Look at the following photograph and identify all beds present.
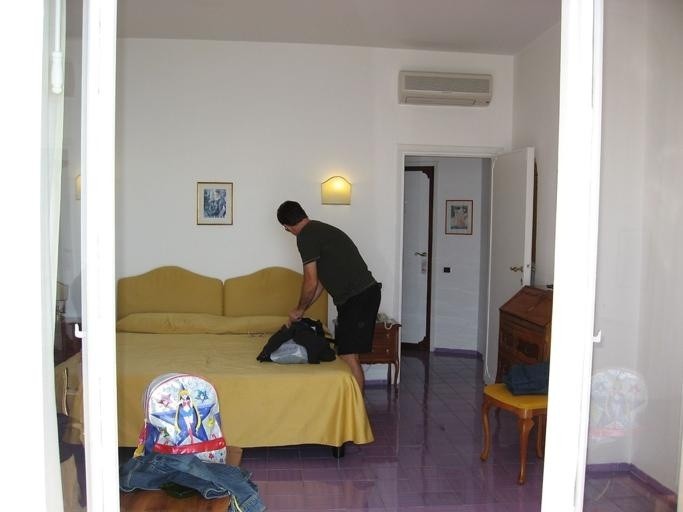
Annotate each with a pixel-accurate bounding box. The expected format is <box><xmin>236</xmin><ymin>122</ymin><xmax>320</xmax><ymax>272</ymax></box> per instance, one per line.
<box><xmin>55</xmin><ymin>266</ymin><xmax>375</xmax><ymax>457</ymax></box>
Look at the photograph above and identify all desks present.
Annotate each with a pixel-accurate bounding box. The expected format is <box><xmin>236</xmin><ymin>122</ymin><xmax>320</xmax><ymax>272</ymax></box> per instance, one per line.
<box><xmin>119</xmin><ymin>445</ymin><xmax>242</xmax><ymax>512</ymax></box>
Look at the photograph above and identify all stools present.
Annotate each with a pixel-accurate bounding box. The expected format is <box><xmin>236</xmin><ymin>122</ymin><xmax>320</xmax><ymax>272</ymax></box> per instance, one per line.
<box><xmin>481</xmin><ymin>384</ymin><xmax>548</xmax><ymax>485</ymax></box>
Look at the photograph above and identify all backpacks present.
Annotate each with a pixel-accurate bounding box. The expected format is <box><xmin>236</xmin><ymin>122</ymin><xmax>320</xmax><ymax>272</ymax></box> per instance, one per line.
<box><xmin>134</xmin><ymin>373</ymin><xmax>227</xmax><ymax>471</ymax></box>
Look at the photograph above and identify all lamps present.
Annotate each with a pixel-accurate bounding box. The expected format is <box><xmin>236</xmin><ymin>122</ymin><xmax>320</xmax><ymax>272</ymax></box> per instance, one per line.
<box><xmin>321</xmin><ymin>176</ymin><xmax>351</xmax><ymax>206</ymax></box>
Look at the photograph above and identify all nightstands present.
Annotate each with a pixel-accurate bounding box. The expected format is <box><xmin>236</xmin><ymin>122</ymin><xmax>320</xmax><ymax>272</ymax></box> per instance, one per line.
<box><xmin>333</xmin><ymin>317</ymin><xmax>401</xmax><ymax>398</ymax></box>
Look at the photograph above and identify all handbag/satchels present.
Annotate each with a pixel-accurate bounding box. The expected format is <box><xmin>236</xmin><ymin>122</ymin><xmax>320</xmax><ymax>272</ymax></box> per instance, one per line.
<box><xmin>501</xmin><ymin>364</ymin><xmax>548</xmax><ymax>395</ymax></box>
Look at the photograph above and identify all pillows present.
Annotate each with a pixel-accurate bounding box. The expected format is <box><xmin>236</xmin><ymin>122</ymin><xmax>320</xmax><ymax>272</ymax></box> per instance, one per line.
<box><xmin>116</xmin><ymin>312</ymin><xmax>333</xmax><ymax>341</ymax></box>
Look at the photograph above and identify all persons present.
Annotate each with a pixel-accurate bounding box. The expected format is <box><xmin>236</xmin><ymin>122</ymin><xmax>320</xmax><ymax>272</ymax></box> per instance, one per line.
<box><xmin>275</xmin><ymin>199</ymin><xmax>383</xmax><ymax>399</ymax></box>
<box><xmin>208</xmin><ymin>191</ymin><xmax>226</xmax><ymax>218</ymax></box>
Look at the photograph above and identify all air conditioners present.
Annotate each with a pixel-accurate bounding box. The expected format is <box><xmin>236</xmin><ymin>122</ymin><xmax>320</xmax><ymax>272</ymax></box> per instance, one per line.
<box><xmin>397</xmin><ymin>72</ymin><xmax>496</xmax><ymax>106</ymax></box>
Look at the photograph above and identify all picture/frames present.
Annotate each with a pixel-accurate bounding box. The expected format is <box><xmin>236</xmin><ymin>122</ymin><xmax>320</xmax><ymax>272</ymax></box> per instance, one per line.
<box><xmin>445</xmin><ymin>199</ymin><xmax>473</xmax><ymax>236</ymax></box>
<box><xmin>196</xmin><ymin>181</ymin><xmax>234</xmax><ymax>225</ymax></box>
